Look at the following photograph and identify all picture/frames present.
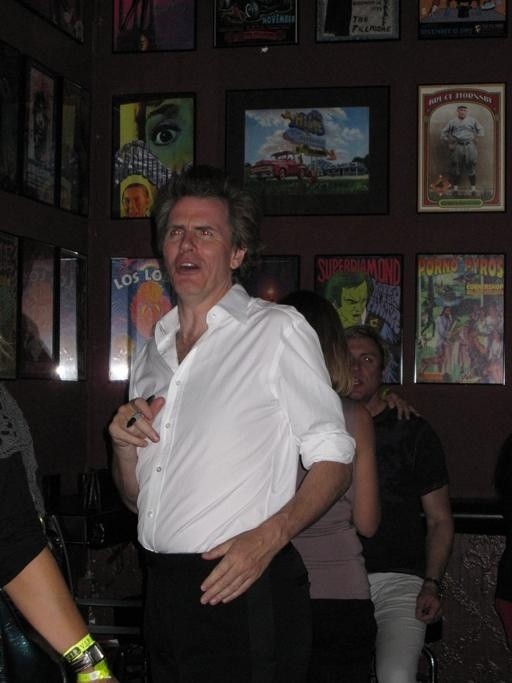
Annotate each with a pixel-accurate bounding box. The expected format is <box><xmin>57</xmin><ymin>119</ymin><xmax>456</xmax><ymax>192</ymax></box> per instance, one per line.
<box><xmin>21</xmin><ymin>54</ymin><xmax>57</xmax><ymax>210</ymax></box>
<box><xmin>222</xmin><ymin>83</ymin><xmax>394</xmax><ymax>219</ymax></box>
<box><xmin>411</xmin><ymin>250</ymin><xmax>507</xmax><ymax>386</ymax></box>
<box><xmin>414</xmin><ymin>81</ymin><xmax>507</xmax><ymax>217</ymax></box>
<box><xmin>58</xmin><ymin>245</ymin><xmax>90</xmax><ymax>382</ymax></box>
<box><xmin>228</xmin><ymin>253</ymin><xmax>303</xmax><ymax>297</ymax></box>
<box><xmin>57</xmin><ymin>72</ymin><xmax>94</xmax><ymax>220</ymax></box>
<box><xmin>212</xmin><ymin>2</ymin><xmax>300</xmax><ymax>50</ymax></box>
<box><xmin>19</xmin><ymin>234</ymin><xmax>57</xmax><ymax>382</ymax></box>
<box><xmin>107</xmin><ymin>89</ymin><xmax>199</xmax><ymax>222</ymax></box>
<box><xmin>416</xmin><ymin>2</ymin><xmax>510</xmax><ymax>42</ymax></box>
<box><xmin>312</xmin><ymin>252</ymin><xmax>407</xmax><ymax>386</ymax></box>
<box><xmin>106</xmin><ymin>255</ymin><xmax>179</xmax><ymax>385</ymax></box>
<box><xmin>314</xmin><ymin>2</ymin><xmax>403</xmax><ymax>44</ymax></box>
<box><xmin>110</xmin><ymin>2</ymin><xmax>198</xmax><ymax>55</ymax></box>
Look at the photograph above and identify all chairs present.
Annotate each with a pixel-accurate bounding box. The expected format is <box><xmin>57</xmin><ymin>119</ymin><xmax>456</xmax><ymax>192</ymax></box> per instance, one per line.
<box><xmin>41</xmin><ymin>469</ymin><xmax>442</xmax><ymax>683</ymax></box>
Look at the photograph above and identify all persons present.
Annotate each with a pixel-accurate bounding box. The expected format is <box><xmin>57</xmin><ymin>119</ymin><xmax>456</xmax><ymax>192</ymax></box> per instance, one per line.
<box><xmin>0</xmin><ymin>384</ymin><xmax>120</xmax><ymax>682</ymax></box>
<box><xmin>327</xmin><ymin>270</ymin><xmax>396</xmax><ymax>381</ymax></box>
<box><xmin>434</xmin><ymin>286</ymin><xmax>505</xmax><ymax>384</ymax></box>
<box><xmin>107</xmin><ymin>176</ymin><xmax>358</xmax><ymax>683</ymax></box>
<box><xmin>490</xmin><ymin>429</ymin><xmax>511</xmax><ymax>651</ymax></box>
<box><xmin>341</xmin><ymin>324</ymin><xmax>455</xmax><ymax>682</ymax></box>
<box><xmin>441</xmin><ymin>105</ymin><xmax>486</xmax><ymax>198</ymax></box>
<box><xmin>117</xmin><ymin>99</ymin><xmax>194</xmax><ymax>216</ymax></box>
<box><xmin>278</xmin><ymin>291</ymin><xmax>421</xmax><ymax>683</ymax></box>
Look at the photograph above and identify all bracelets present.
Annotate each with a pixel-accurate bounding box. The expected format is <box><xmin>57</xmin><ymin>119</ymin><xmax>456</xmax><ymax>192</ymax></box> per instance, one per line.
<box><xmin>71</xmin><ymin>641</ymin><xmax>105</xmax><ymax>671</ymax></box>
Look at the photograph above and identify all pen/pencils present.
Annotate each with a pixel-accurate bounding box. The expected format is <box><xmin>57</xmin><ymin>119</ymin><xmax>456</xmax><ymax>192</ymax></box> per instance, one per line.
<box><xmin>126</xmin><ymin>395</ymin><xmax>155</xmax><ymax>428</ymax></box>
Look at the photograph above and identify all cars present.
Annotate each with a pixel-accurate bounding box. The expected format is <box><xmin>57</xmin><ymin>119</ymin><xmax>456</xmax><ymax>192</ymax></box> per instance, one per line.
<box><xmin>253</xmin><ymin>150</ymin><xmax>308</xmax><ymax>181</ymax></box>
